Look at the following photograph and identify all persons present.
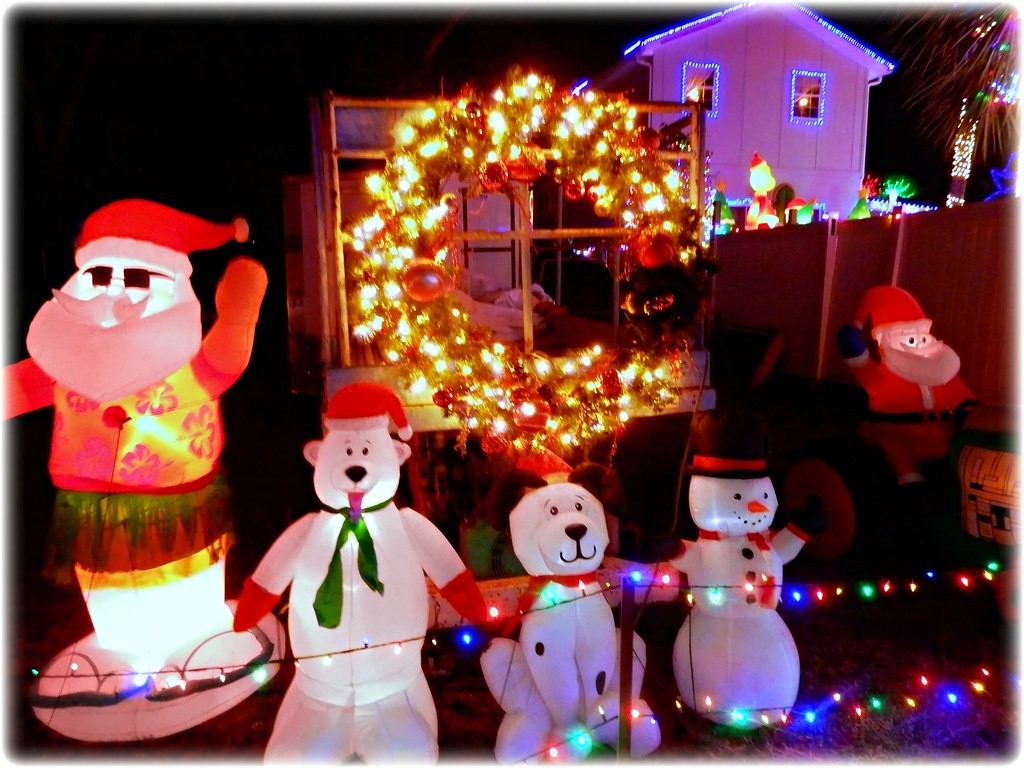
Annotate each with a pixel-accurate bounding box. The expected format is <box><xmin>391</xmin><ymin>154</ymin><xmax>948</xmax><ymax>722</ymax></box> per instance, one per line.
<box><xmin>6</xmin><ymin>201</ymin><xmax>267</xmax><ymax>689</ymax></box>
<box><xmin>837</xmin><ymin>285</ymin><xmax>975</xmax><ymax>504</ymax></box>
